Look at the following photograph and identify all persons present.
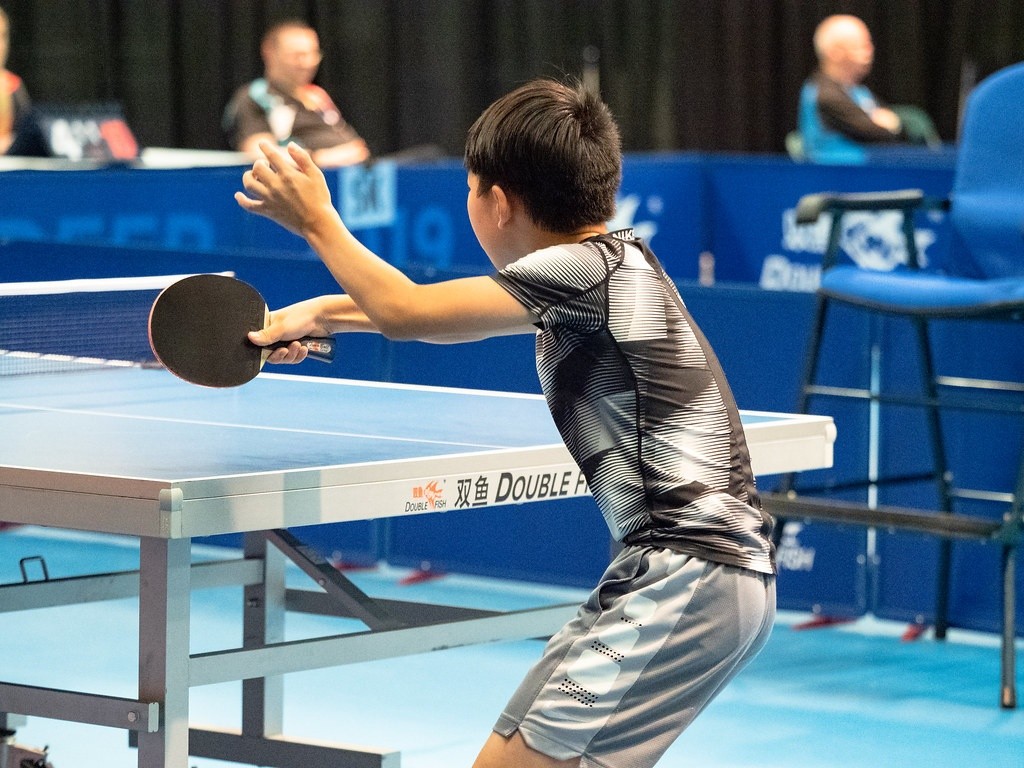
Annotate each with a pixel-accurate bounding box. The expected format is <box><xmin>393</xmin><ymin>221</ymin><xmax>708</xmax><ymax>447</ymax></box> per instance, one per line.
<box><xmin>799</xmin><ymin>14</ymin><xmax>910</xmax><ymax>162</ymax></box>
<box><xmin>232</xmin><ymin>80</ymin><xmax>776</xmax><ymax>768</ymax></box>
<box><xmin>0</xmin><ymin>8</ymin><xmax>32</xmax><ymax>155</ymax></box>
<box><xmin>224</xmin><ymin>18</ymin><xmax>370</xmax><ymax>169</ymax></box>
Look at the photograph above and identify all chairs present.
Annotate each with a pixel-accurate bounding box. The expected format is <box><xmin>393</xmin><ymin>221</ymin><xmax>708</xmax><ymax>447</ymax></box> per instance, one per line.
<box><xmin>760</xmin><ymin>61</ymin><xmax>1024</xmax><ymax>711</ymax></box>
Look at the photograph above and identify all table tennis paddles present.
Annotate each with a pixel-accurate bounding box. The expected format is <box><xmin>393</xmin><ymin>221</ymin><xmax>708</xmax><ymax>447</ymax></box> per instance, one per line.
<box><xmin>146</xmin><ymin>270</ymin><xmax>337</xmax><ymax>389</ymax></box>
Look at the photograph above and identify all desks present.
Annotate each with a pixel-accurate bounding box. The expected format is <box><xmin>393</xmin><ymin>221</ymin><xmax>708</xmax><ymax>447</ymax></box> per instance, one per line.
<box><xmin>0</xmin><ymin>271</ymin><xmax>840</xmax><ymax>768</ymax></box>
